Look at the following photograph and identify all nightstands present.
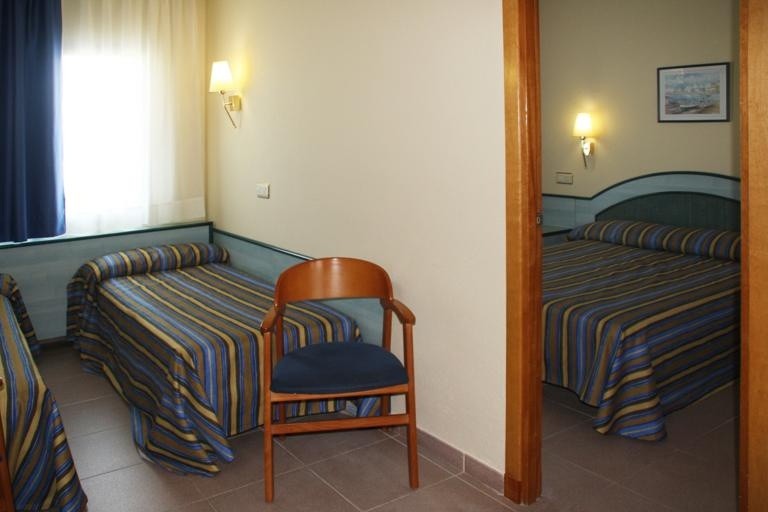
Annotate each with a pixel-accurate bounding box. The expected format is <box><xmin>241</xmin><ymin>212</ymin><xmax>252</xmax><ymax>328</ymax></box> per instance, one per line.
<box><xmin>542</xmin><ymin>225</ymin><xmax>572</xmax><ymax>245</ymax></box>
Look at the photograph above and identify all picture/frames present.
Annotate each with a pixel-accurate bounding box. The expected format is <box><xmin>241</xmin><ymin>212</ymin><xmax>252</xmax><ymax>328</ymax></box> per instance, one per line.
<box><xmin>657</xmin><ymin>61</ymin><xmax>730</xmax><ymax>123</ymax></box>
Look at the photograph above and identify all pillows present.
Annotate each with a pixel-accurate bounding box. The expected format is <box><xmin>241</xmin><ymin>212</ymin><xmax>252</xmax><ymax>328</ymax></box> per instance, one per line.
<box><xmin>567</xmin><ymin>219</ymin><xmax>741</xmax><ymax>261</ymax></box>
<box><xmin>79</xmin><ymin>241</ymin><xmax>230</xmax><ymax>282</ymax></box>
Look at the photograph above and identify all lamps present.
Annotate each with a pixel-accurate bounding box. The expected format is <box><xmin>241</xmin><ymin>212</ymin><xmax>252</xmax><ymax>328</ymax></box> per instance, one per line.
<box><xmin>209</xmin><ymin>60</ymin><xmax>240</xmax><ymax>129</ymax></box>
<box><xmin>572</xmin><ymin>111</ymin><xmax>593</xmax><ymax>168</ymax></box>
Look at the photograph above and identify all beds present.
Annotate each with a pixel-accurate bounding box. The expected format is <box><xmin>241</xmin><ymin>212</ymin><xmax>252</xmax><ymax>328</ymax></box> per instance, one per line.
<box><xmin>65</xmin><ymin>242</ymin><xmax>390</xmax><ymax>479</ymax></box>
<box><xmin>541</xmin><ymin>190</ymin><xmax>742</xmax><ymax>442</ymax></box>
<box><xmin>0</xmin><ymin>273</ymin><xmax>87</xmax><ymax>511</ymax></box>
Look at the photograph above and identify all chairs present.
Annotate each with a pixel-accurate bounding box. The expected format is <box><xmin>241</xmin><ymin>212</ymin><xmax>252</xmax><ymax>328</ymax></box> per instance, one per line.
<box><xmin>259</xmin><ymin>255</ymin><xmax>419</xmax><ymax>502</ymax></box>
